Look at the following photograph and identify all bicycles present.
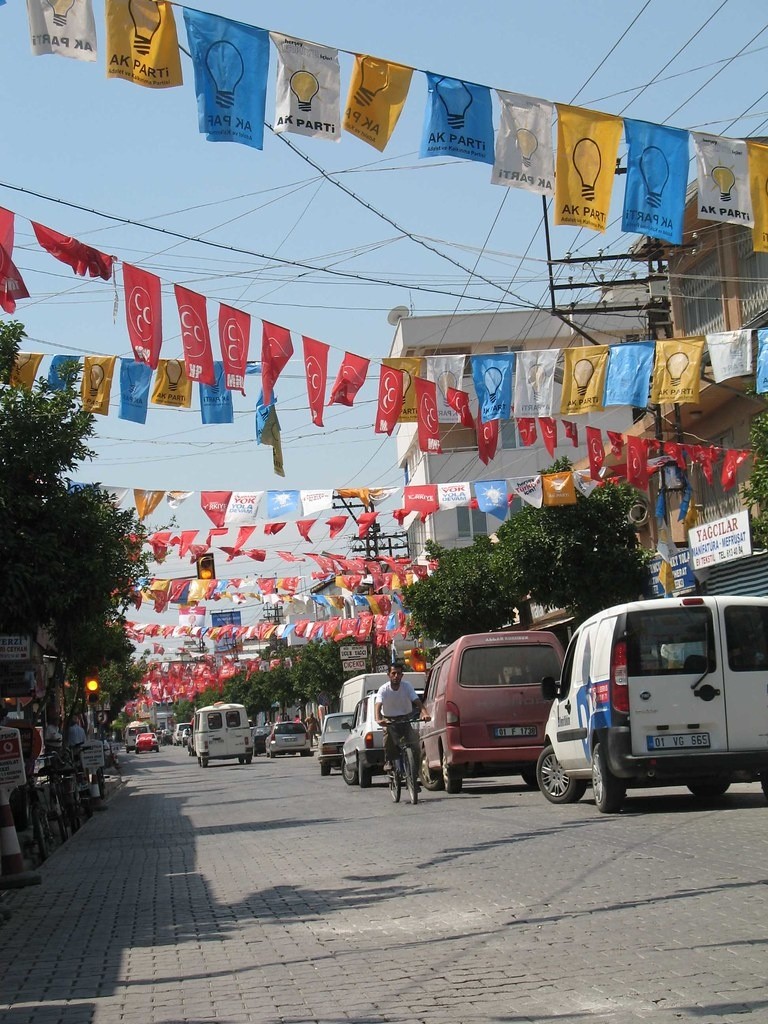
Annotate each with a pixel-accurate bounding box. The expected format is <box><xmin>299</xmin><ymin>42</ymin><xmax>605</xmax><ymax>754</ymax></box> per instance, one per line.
<box><xmin>21</xmin><ymin>738</ymin><xmax>110</xmax><ymax>865</ymax></box>
<box><xmin>379</xmin><ymin>718</ymin><xmax>429</xmax><ymax>806</ymax></box>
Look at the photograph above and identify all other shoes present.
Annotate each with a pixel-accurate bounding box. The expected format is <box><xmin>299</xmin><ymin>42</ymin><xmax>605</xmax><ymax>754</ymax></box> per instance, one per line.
<box><xmin>383</xmin><ymin>761</ymin><xmax>393</xmax><ymax>771</ymax></box>
<box><xmin>417</xmin><ymin>784</ymin><xmax>422</xmax><ymax>793</ymax></box>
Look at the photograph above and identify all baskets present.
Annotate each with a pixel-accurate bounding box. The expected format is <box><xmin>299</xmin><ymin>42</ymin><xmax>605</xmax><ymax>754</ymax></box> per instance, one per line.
<box><xmin>388</xmin><ymin>721</ymin><xmax>419</xmax><ymax>745</ymax></box>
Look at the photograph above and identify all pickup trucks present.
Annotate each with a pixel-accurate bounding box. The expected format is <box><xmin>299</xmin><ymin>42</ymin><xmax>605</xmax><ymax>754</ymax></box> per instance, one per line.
<box><xmin>133</xmin><ymin>733</ymin><xmax>160</xmax><ymax>753</ymax></box>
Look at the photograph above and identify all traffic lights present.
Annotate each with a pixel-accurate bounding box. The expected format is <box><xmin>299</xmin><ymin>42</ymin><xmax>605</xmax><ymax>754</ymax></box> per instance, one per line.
<box><xmin>86</xmin><ymin>675</ymin><xmax>100</xmax><ymax>707</ymax></box>
<box><xmin>197</xmin><ymin>553</ymin><xmax>216</xmax><ymax>579</ymax></box>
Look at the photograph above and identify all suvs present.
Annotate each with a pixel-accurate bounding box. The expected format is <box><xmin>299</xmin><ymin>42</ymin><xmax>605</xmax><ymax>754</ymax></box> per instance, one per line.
<box><xmin>340</xmin><ymin>691</ymin><xmax>425</xmax><ymax>788</ymax></box>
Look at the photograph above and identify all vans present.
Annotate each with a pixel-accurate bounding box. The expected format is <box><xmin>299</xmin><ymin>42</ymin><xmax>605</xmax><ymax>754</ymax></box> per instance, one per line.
<box><xmin>339</xmin><ymin>672</ymin><xmax>427</xmax><ymax>715</ymax></box>
<box><xmin>536</xmin><ymin>595</ymin><xmax>768</xmax><ymax>813</ymax></box>
<box><xmin>418</xmin><ymin>630</ymin><xmax>569</xmax><ymax>794</ymax></box>
<box><xmin>189</xmin><ymin>704</ymin><xmax>254</xmax><ymax>768</ymax></box>
<box><xmin>124</xmin><ymin>724</ymin><xmax>152</xmax><ymax>754</ymax></box>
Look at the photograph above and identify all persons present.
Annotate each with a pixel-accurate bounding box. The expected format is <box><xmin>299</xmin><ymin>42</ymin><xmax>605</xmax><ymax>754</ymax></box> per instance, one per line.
<box><xmin>45</xmin><ymin>714</ymin><xmax>86</xmax><ymax>767</ymax></box>
<box><xmin>375</xmin><ymin>663</ymin><xmax>431</xmax><ymax>793</ymax></box>
<box><xmin>293</xmin><ymin>712</ymin><xmax>322</xmax><ymax>745</ymax></box>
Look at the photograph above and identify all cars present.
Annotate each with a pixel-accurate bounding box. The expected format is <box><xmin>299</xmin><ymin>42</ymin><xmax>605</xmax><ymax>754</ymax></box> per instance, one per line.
<box><xmin>264</xmin><ymin>721</ymin><xmax>312</xmax><ymax>758</ymax></box>
<box><xmin>318</xmin><ymin>712</ymin><xmax>356</xmax><ymax>776</ymax></box>
<box><xmin>163</xmin><ymin>722</ymin><xmax>196</xmax><ymax>757</ymax></box>
<box><xmin>252</xmin><ymin>726</ymin><xmax>273</xmax><ymax>758</ymax></box>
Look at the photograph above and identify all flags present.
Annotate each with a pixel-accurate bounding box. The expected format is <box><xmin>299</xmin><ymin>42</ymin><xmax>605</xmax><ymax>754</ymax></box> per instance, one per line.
<box><xmin>692</xmin><ymin>131</ymin><xmax>768</xmax><ymax>252</ymax></box>
<box><xmin>268</xmin><ymin>32</ymin><xmax>341</xmax><ymax>142</ymax></box>
<box><xmin>26</xmin><ymin>0</ymin><xmax>97</xmax><ymax>61</ymax></box>
<box><xmin>418</xmin><ymin>74</ymin><xmax>495</xmax><ymax>165</ymax></box>
<box><xmin>105</xmin><ymin>0</ymin><xmax>183</xmax><ymax>88</ymax></box>
<box><xmin>554</xmin><ymin>103</ymin><xmax>623</xmax><ymax>234</ymax></box>
<box><xmin>621</xmin><ymin>118</ymin><xmax>690</xmax><ymax>245</ymax></box>
<box><xmin>0</xmin><ymin>208</ymin><xmax>768</xmax><ymax>716</ymax></box>
<box><xmin>490</xmin><ymin>91</ymin><xmax>554</xmax><ymax>197</ymax></box>
<box><xmin>182</xmin><ymin>6</ymin><xmax>270</xmax><ymax>150</ymax></box>
<box><xmin>340</xmin><ymin>53</ymin><xmax>413</xmax><ymax>152</ymax></box>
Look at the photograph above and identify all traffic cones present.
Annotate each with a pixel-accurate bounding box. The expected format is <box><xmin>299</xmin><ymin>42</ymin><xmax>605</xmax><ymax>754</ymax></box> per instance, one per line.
<box><xmin>114</xmin><ymin>755</ymin><xmax>122</xmax><ymax>767</ymax></box>
